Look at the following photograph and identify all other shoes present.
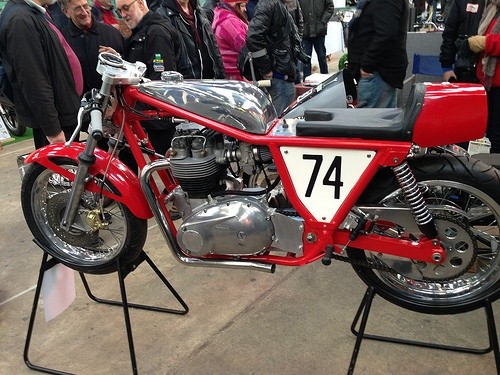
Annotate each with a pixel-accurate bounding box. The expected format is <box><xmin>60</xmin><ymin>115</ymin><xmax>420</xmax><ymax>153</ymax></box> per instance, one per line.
<box><xmin>100</xmin><ymin>211</ymin><xmax>112</xmax><ymax>224</ymax></box>
<box><xmin>91</xmin><ymin>236</ymin><xmax>104</xmax><ymax>247</ymax></box>
<box><xmin>96</xmin><ymin>194</ymin><xmax>114</xmax><ymax>208</ymax></box>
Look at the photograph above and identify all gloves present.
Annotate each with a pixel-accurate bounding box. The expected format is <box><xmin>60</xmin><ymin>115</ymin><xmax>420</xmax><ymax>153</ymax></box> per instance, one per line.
<box><xmin>468</xmin><ymin>35</ymin><xmax>486</xmax><ymax>53</ymax></box>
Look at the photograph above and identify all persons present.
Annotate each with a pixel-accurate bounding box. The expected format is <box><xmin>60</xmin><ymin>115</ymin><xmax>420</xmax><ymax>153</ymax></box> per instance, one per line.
<box><xmin>298</xmin><ymin>0</ymin><xmax>335</xmax><ymax>83</ymax></box>
<box><xmin>0</xmin><ymin>0</ymin><xmax>84</xmax><ymax>150</ymax></box>
<box><xmin>90</xmin><ymin>0</ymin><xmax>313</xmax><ymax>116</ymax></box>
<box><xmin>467</xmin><ymin>0</ymin><xmax>500</xmax><ymax>153</ymax></box>
<box><xmin>347</xmin><ymin>0</ymin><xmax>410</xmax><ymax>108</ymax></box>
<box><xmin>57</xmin><ymin>0</ymin><xmax>126</xmax><ymax>133</ymax></box>
<box><xmin>438</xmin><ymin>0</ymin><xmax>486</xmax><ymax>82</ymax></box>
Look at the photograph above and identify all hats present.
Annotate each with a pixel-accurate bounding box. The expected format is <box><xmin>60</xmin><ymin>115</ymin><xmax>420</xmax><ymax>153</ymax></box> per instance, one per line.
<box><xmin>221</xmin><ymin>0</ymin><xmax>248</xmax><ymax>7</ymax></box>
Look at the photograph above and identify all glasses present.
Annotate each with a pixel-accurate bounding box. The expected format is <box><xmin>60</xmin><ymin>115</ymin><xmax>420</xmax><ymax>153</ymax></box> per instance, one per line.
<box><xmin>115</xmin><ymin>0</ymin><xmax>137</xmax><ymax>15</ymax></box>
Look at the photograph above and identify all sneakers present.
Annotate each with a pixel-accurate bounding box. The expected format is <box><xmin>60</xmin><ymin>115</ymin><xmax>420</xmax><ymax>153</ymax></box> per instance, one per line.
<box><xmin>168</xmin><ymin>203</ymin><xmax>182</xmax><ymax>220</ymax></box>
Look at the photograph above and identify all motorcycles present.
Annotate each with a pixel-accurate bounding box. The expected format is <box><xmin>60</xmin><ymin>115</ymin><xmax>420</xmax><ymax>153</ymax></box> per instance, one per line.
<box><xmin>18</xmin><ymin>46</ymin><xmax>500</xmax><ymax>316</ymax></box>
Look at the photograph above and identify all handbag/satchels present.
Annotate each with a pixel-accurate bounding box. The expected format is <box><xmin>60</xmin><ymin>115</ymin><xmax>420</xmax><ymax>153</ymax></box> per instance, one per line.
<box><xmin>454</xmin><ymin>35</ymin><xmax>478</xmax><ymax>83</ymax></box>
<box><xmin>236</xmin><ymin>46</ymin><xmax>261</xmax><ymax>81</ymax></box>
